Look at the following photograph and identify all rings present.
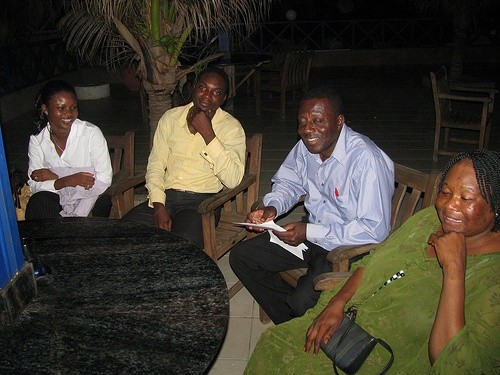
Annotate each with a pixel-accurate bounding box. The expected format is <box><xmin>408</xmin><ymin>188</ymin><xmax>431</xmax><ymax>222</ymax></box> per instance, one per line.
<box><xmin>35</xmin><ymin>177</ymin><xmax>37</xmax><ymax>179</ymax></box>
<box><xmin>87</xmin><ymin>185</ymin><xmax>89</xmax><ymax>188</ymax></box>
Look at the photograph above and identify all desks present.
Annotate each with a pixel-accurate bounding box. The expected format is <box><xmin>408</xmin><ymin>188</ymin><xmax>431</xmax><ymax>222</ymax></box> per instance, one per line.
<box><xmin>211</xmin><ymin>53</ymin><xmax>273</xmax><ymax>97</ymax></box>
<box><xmin>0</xmin><ymin>220</ymin><xmax>231</xmax><ymax>375</ymax></box>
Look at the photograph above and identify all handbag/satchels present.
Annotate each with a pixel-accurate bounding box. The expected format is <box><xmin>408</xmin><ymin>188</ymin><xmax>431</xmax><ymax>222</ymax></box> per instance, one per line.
<box><xmin>318</xmin><ymin>306</ymin><xmax>395</xmax><ymax>375</ymax></box>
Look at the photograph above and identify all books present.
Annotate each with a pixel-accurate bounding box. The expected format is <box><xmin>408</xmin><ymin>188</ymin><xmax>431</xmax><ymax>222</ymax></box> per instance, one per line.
<box><xmin>232</xmin><ymin>221</ymin><xmax>309</xmax><ymax>260</ymax></box>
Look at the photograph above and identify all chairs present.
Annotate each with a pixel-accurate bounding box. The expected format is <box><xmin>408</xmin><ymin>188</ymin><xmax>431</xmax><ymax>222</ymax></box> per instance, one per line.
<box><xmin>251</xmin><ymin>162</ymin><xmax>443</xmax><ymax>324</ymax></box>
<box><xmin>104</xmin><ymin>132</ymin><xmax>262</xmax><ymax>299</ymax></box>
<box><xmin>177</xmin><ymin>43</ymin><xmax>314</xmax><ymax>121</ymax></box>
<box><xmin>87</xmin><ymin>131</ymin><xmax>136</xmax><ymax>218</ymax></box>
<box><xmin>429</xmin><ymin>64</ymin><xmax>498</xmax><ymax>162</ymax></box>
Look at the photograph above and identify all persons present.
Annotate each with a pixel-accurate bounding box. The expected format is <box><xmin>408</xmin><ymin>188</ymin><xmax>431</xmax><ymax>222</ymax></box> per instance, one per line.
<box><xmin>25</xmin><ymin>84</ymin><xmax>114</xmax><ymax>258</ymax></box>
<box><xmin>122</xmin><ymin>67</ymin><xmax>247</xmax><ymax>248</ymax></box>
<box><xmin>242</xmin><ymin>152</ymin><xmax>500</xmax><ymax>375</ymax></box>
<box><xmin>230</xmin><ymin>88</ymin><xmax>395</xmax><ymax>326</ymax></box>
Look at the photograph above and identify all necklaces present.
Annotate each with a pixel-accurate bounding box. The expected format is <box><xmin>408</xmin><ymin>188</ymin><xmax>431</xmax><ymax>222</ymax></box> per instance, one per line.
<box><xmin>51</xmin><ymin>131</ymin><xmax>64</xmax><ymax>152</ymax></box>
